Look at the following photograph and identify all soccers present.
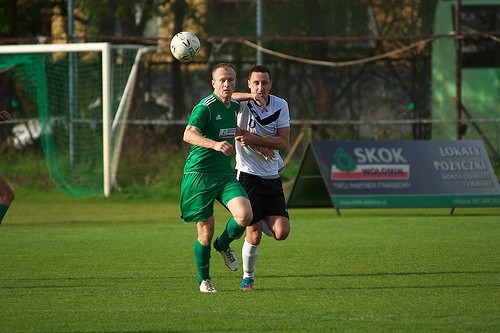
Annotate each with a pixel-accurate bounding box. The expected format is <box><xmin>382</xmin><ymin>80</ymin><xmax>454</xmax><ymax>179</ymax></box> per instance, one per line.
<box><xmin>169</xmin><ymin>31</ymin><xmax>201</xmax><ymax>64</ymax></box>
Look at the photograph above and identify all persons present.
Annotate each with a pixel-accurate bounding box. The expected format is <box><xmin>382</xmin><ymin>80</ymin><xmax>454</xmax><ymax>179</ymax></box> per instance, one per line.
<box><xmin>180</xmin><ymin>63</ymin><xmax>275</xmax><ymax>293</ymax></box>
<box><xmin>231</xmin><ymin>65</ymin><xmax>290</xmax><ymax>291</ymax></box>
<box><xmin>0</xmin><ymin>111</ymin><xmax>15</xmax><ymax>225</ymax></box>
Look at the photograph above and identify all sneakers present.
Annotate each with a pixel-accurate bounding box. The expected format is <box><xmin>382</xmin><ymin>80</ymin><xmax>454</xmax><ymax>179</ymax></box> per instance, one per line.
<box><xmin>199</xmin><ymin>279</ymin><xmax>217</xmax><ymax>293</ymax></box>
<box><xmin>240</xmin><ymin>277</ymin><xmax>254</xmax><ymax>291</ymax></box>
<box><xmin>213</xmin><ymin>237</ymin><xmax>238</xmax><ymax>271</ymax></box>
